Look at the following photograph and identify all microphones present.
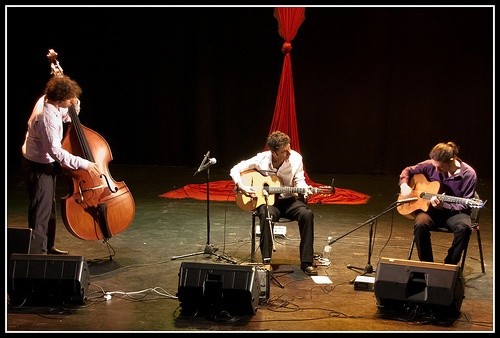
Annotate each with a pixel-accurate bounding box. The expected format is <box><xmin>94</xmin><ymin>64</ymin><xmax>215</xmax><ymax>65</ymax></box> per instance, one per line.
<box><xmin>395</xmin><ymin>197</ymin><xmax>418</xmax><ymax>203</ymax></box>
<box><xmin>192</xmin><ymin>158</ymin><xmax>216</xmax><ymax>176</ymax></box>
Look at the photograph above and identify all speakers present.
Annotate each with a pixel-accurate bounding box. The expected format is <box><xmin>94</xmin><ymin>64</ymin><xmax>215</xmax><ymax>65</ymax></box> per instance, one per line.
<box><xmin>8</xmin><ymin>254</ymin><xmax>91</xmax><ymax>306</ymax></box>
<box><xmin>374</xmin><ymin>257</ymin><xmax>466</xmax><ymax>326</ymax></box>
<box><xmin>178</xmin><ymin>263</ymin><xmax>261</xmax><ymax>323</ymax></box>
<box><xmin>8</xmin><ymin>227</ymin><xmax>47</xmax><ymax>255</ymax></box>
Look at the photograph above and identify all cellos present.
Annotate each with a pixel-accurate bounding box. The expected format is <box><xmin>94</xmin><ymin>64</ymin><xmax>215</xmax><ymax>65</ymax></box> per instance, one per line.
<box><xmin>47</xmin><ymin>48</ymin><xmax>135</xmax><ymax>259</ymax></box>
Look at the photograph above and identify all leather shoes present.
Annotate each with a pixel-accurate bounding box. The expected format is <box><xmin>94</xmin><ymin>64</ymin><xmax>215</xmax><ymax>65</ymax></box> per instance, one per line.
<box><xmin>301</xmin><ymin>262</ymin><xmax>317</xmax><ymax>274</ymax></box>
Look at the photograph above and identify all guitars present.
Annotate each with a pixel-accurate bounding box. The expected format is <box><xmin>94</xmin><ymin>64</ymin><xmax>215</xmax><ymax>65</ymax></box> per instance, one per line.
<box><xmin>235</xmin><ymin>169</ymin><xmax>335</xmax><ymax>212</ymax></box>
<box><xmin>396</xmin><ymin>173</ymin><xmax>487</xmax><ymax>220</ymax></box>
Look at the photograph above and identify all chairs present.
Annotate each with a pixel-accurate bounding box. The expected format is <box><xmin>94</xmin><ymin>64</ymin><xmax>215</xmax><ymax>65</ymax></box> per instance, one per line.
<box><xmin>251</xmin><ymin>195</ymin><xmax>307</xmax><ymax>262</ymax></box>
<box><xmin>407</xmin><ymin>192</ymin><xmax>485</xmax><ymax>274</ymax></box>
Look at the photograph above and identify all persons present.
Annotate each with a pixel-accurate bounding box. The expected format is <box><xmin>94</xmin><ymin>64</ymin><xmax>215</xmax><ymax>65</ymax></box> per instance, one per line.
<box><xmin>22</xmin><ymin>76</ymin><xmax>103</xmax><ymax>255</ymax></box>
<box><xmin>229</xmin><ymin>131</ymin><xmax>318</xmax><ymax>277</ymax></box>
<box><xmin>398</xmin><ymin>142</ymin><xmax>478</xmax><ymax>265</ymax></box>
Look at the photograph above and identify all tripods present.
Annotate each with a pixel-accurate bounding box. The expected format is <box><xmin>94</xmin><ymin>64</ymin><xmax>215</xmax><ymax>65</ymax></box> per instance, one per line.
<box><xmin>170</xmin><ymin>168</ymin><xmax>235</xmax><ymax>262</ymax></box>
<box><xmin>330</xmin><ymin>200</ymin><xmax>400</xmax><ymax>283</ymax></box>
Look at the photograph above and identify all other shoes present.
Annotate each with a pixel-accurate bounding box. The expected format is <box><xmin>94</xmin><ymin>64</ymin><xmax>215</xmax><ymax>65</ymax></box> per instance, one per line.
<box><xmin>48</xmin><ymin>249</ymin><xmax>69</xmax><ymax>254</ymax></box>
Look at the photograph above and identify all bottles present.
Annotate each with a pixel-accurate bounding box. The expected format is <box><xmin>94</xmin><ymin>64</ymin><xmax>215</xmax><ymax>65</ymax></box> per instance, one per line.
<box><xmin>322</xmin><ymin>237</ymin><xmax>333</xmax><ymax>259</ymax></box>
